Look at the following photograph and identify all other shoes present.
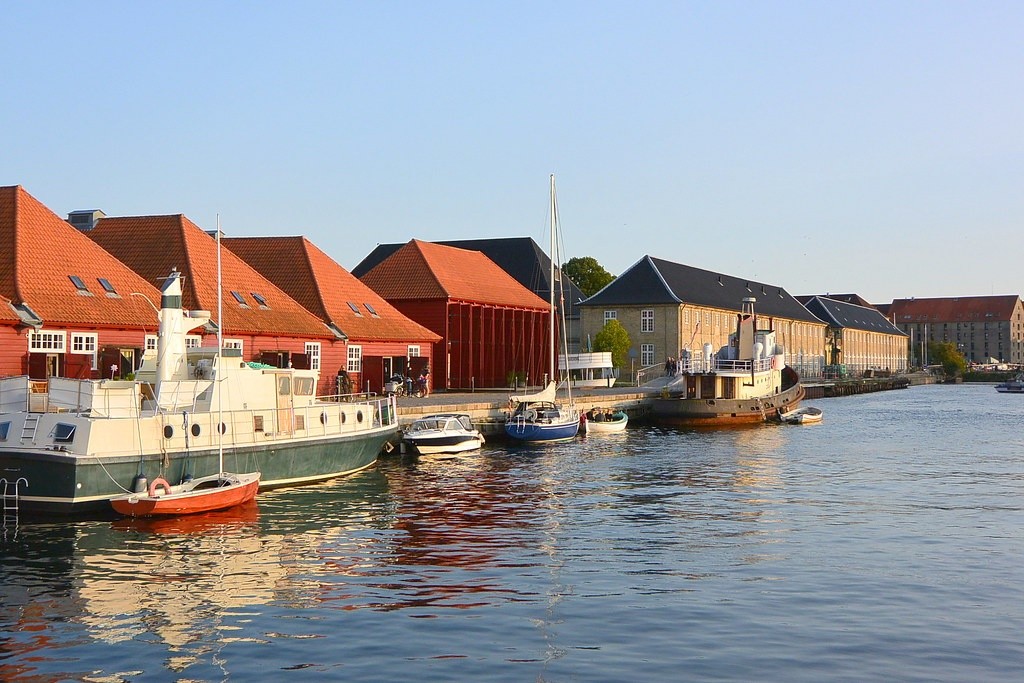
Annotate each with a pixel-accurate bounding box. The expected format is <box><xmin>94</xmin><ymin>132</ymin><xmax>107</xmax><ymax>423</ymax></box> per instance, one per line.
<box><xmin>424</xmin><ymin>395</ymin><xmax>429</xmax><ymax>398</ymax></box>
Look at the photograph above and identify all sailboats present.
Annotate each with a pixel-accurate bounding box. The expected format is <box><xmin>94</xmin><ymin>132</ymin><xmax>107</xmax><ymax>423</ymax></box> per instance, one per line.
<box><xmin>108</xmin><ymin>213</ymin><xmax>263</xmax><ymax>518</ymax></box>
<box><xmin>505</xmin><ymin>174</ymin><xmax>586</xmax><ymax>447</ymax></box>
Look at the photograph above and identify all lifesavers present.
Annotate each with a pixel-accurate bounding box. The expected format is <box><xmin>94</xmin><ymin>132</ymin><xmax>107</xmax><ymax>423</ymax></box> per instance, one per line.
<box><xmin>148</xmin><ymin>477</ymin><xmax>172</xmax><ymax>496</ymax></box>
<box><xmin>771</xmin><ymin>358</ymin><xmax>775</xmax><ymax>368</ymax></box>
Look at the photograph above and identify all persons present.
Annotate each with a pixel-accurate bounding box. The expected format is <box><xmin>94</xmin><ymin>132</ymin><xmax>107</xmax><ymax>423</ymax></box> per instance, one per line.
<box><xmin>419</xmin><ymin>364</ymin><xmax>431</xmax><ymax>398</ymax></box>
<box><xmin>585</xmin><ymin>408</ymin><xmax>596</xmax><ymax>423</ymax></box>
<box><xmin>663</xmin><ymin>356</ymin><xmax>678</xmax><ymax>378</ymax></box>
<box><xmin>594</xmin><ymin>411</ymin><xmax>605</xmax><ymax>422</ymax></box>
<box><xmin>335</xmin><ymin>364</ymin><xmax>354</xmax><ymax>402</ymax></box>
<box><xmin>406</xmin><ymin>366</ymin><xmax>416</xmax><ymax>399</ymax></box>
<box><xmin>605</xmin><ymin>411</ymin><xmax>612</xmax><ymax>422</ymax></box>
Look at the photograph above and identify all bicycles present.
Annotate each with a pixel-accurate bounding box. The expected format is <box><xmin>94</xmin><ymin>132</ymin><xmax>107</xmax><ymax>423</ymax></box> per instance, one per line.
<box><xmin>397</xmin><ymin>380</ymin><xmax>428</xmax><ymax>397</ymax></box>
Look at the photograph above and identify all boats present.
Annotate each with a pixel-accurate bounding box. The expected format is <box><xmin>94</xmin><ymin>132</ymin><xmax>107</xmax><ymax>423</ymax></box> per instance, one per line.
<box><xmin>0</xmin><ymin>273</ymin><xmax>399</xmax><ymax>521</ymax></box>
<box><xmin>638</xmin><ymin>297</ymin><xmax>806</xmax><ymax>427</ymax></box>
<box><xmin>994</xmin><ymin>378</ymin><xmax>1024</xmax><ymax>394</ymax></box>
<box><xmin>780</xmin><ymin>406</ymin><xmax>823</xmax><ymax>425</ymax></box>
<box><xmin>579</xmin><ymin>407</ymin><xmax>629</xmax><ymax>437</ymax></box>
<box><xmin>401</xmin><ymin>413</ymin><xmax>485</xmax><ymax>457</ymax></box>
